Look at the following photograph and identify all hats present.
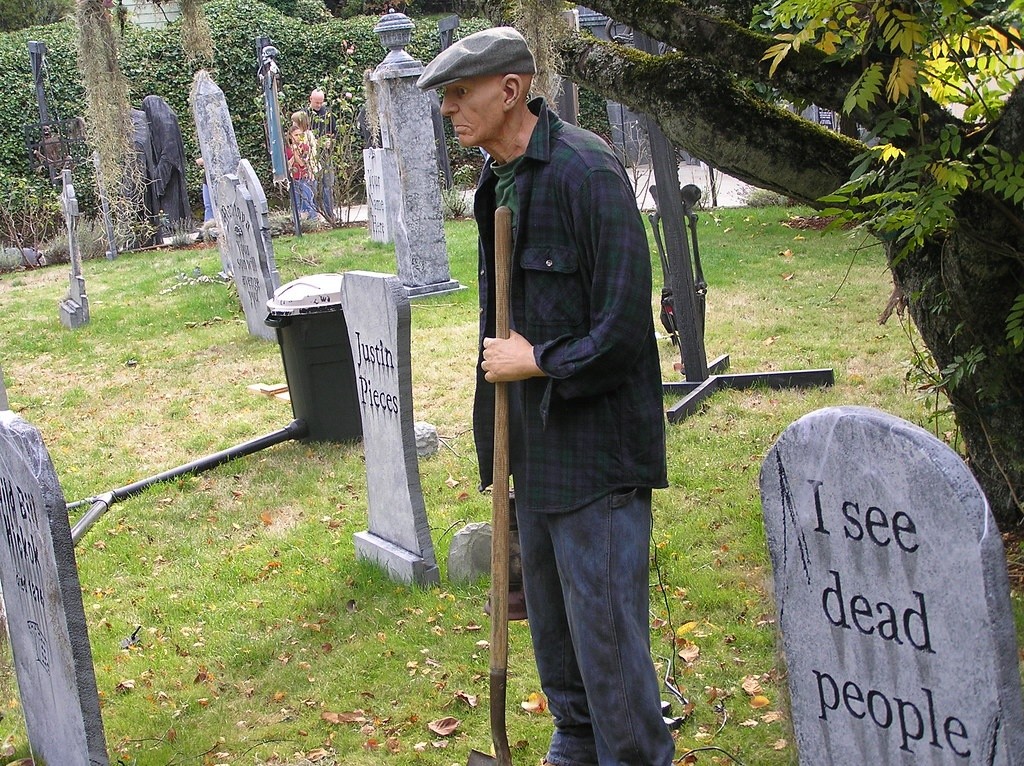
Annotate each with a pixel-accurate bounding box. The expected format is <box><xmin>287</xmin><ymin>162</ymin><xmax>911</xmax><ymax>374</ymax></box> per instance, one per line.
<box><xmin>416</xmin><ymin>26</ymin><xmax>536</xmax><ymax>92</ymax></box>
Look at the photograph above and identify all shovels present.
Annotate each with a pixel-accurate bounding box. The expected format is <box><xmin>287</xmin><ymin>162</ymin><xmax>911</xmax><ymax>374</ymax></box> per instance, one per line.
<box><xmin>464</xmin><ymin>202</ymin><xmax>516</xmax><ymax>765</ymax></box>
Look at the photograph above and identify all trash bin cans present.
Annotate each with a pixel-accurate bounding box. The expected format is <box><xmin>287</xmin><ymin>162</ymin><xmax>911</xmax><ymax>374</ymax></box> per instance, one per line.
<box><xmin>265</xmin><ymin>273</ymin><xmax>364</xmax><ymax>443</ymax></box>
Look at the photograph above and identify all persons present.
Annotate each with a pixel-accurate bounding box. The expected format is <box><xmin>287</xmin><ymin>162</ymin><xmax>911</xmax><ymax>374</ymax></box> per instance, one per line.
<box><xmin>417</xmin><ymin>25</ymin><xmax>676</xmax><ymax>766</ymax></box>
<box><xmin>118</xmin><ymin>95</ymin><xmax>192</xmax><ymax>249</ymax></box>
<box><xmin>286</xmin><ymin>89</ymin><xmax>343</xmax><ymax>226</ymax></box>
<box><xmin>196</xmin><ymin>157</ymin><xmax>214</xmax><ymax>242</ymax></box>
